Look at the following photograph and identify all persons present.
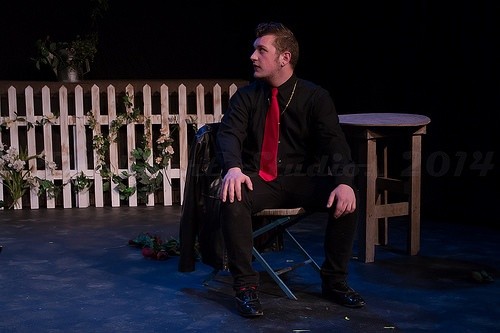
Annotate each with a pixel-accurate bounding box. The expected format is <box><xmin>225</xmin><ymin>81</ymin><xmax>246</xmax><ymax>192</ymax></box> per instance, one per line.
<box><xmin>216</xmin><ymin>22</ymin><xmax>366</xmax><ymax>317</ymax></box>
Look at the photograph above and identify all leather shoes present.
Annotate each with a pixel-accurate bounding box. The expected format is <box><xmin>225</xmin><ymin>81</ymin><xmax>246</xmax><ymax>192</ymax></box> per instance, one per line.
<box><xmin>322</xmin><ymin>284</ymin><xmax>366</xmax><ymax>306</ymax></box>
<box><xmin>232</xmin><ymin>285</ymin><xmax>265</xmax><ymax>316</ymax></box>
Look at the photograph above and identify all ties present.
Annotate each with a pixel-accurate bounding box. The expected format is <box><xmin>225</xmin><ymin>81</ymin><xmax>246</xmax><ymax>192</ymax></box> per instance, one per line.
<box><xmin>259</xmin><ymin>87</ymin><xmax>279</xmax><ymax>182</ymax></box>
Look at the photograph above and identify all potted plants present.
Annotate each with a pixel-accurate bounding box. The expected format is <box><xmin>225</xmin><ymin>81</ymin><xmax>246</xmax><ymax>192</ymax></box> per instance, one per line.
<box><xmin>30</xmin><ymin>34</ymin><xmax>98</xmax><ymax>83</ymax></box>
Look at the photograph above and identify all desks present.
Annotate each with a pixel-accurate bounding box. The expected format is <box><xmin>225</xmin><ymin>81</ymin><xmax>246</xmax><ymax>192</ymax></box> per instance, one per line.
<box><xmin>339</xmin><ymin>112</ymin><xmax>432</xmax><ymax>264</ymax></box>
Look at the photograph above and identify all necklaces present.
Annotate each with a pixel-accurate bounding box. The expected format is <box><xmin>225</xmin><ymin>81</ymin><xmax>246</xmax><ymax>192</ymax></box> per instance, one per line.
<box><xmin>268</xmin><ymin>81</ymin><xmax>297</xmax><ymax>116</ymax></box>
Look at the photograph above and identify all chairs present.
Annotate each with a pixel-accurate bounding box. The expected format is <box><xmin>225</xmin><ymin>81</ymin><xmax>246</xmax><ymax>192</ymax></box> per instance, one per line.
<box><xmin>176</xmin><ymin>122</ymin><xmax>323</xmax><ymax>301</ymax></box>
